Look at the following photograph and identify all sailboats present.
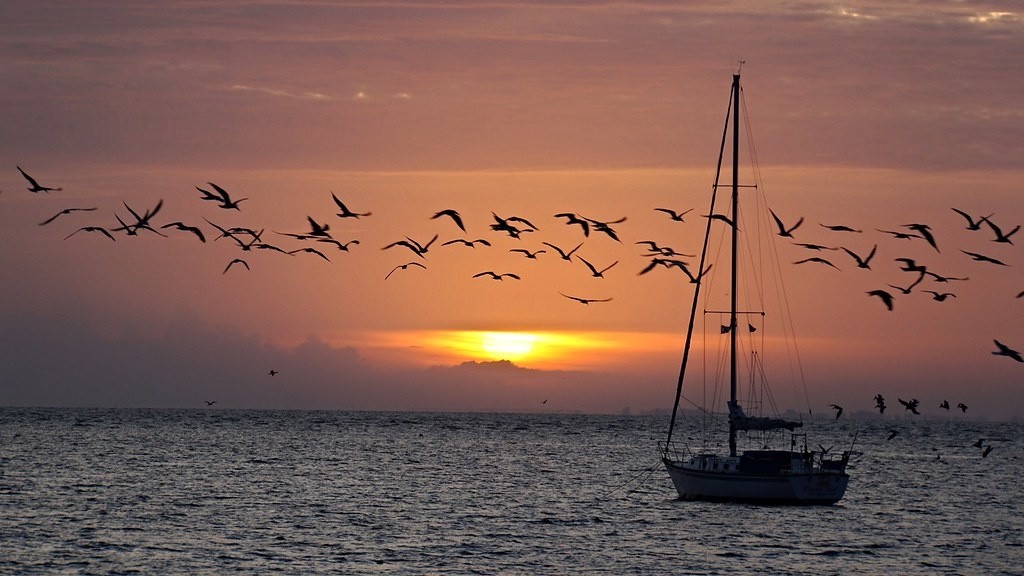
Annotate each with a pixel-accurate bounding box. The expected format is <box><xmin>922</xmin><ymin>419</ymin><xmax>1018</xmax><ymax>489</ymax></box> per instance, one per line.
<box><xmin>657</xmin><ymin>72</ymin><xmax>855</xmax><ymax>505</ymax></box>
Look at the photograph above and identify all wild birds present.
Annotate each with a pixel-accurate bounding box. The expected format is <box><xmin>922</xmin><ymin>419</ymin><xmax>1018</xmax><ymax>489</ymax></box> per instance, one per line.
<box><xmin>306</xmin><ymin>217</ymin><xmax>333</xmax><ymax>239</ymax></box>
<box><xmin>38</xmin><ymin>205</ymin><xmax>98</xmax><ymax>227</ymax></box>
<box><xmin>215</xmin><ymin>228</ymin><xmax>261</xmax><ymax>243</ymax></box>
<box><xmin>225</xmin><ymin>229</ymin><xmax>266</xmax><ymax>251</ymax></box>
<box><xmin>330</xmin><ymin>191</ymin><xmax>373</xmax><ymax>219</ymax></box>
<box><xmin>991</xmin><ymin>338</ymin><xmax>1023</xmax><ymax>362</ymax></box>
<box><xmin>208</xmin><ymin>181</ymin><xmax>248</xmax><ymax>211</ymax></box>
<box><xmin>235</xmin><ymin>243</ymin><xmax>296</xmax><ymax>257</ymax></box>
<box><xmin>289</xmin><ymin>248</ymin><xmax>332</xmax><ymax>263</ymax></box>
<box><xmin>195</xmin><ymin>187</ymin><xmax>225</xmax><ymax>203</ymax></box>
<box><xmin>64</xmin><ymin>227</ymin><xmax>115</xmax><ymax>243</ymax></box>
<box><xmin>162</xmin><ymin>221</ymin><xmax>206</xmax><ymax>243</ymax></box>
<box><xmin>108</xmin><ymin>223</ymin><xmax>168</xmax><ymax>237</ymax></box>
<box><xmin>320</xmin><ymin>240</ymin><xmax>360</xmax><ymax>253</ymax></box>
<box><xmin>111</xmin><ymin>209</ymin><xmax>150</xmax><ymax>235</ymax></box>
<box><xmin>123</xmin><ymin>199</ymin><xmax>163</xmax><ymax>227</ymax></box>
<box><xmin>381</xmin><ymin>198</ymin><xmax>1023</xmax><ymax>310</ymax></box>
<box><xmin>829</xmin><ymin>392</ymin><xmax>994</xmax><ymax>465</ymax></box>
<box><xmin>17</xmin><ymin>167</ymin><xmax>64</xmax><ymax>195</ymax></box>
<box><xmin>272</xmin><ymin>229</ymin><xmax>326</xmax><ymax>241</ymax></box>
<box><xmin>203</xmin><ymin>215</ymin><xmax>258</xmax><ymax>236</ymax></box>
<box><xmin>223</xmin><ymin>258</ymin><xmax>250</xmax><ymax>273</ymax></box>
<box><xmin>288</xmin><ymin>248</ymin><xmax>331</xmax><ymax>262</ymax></box>
